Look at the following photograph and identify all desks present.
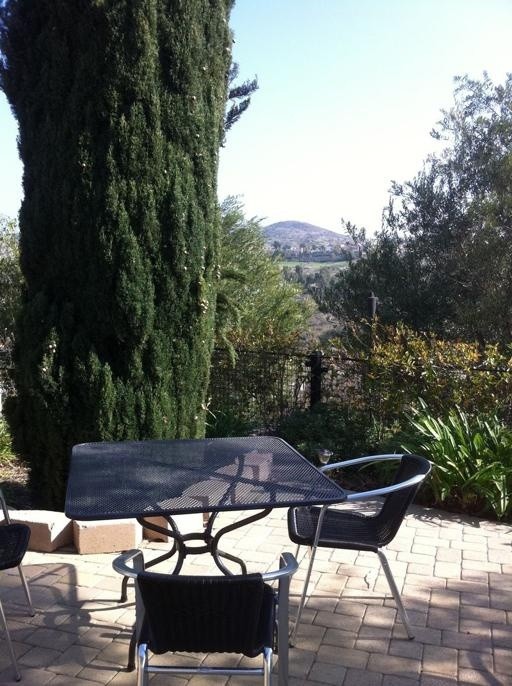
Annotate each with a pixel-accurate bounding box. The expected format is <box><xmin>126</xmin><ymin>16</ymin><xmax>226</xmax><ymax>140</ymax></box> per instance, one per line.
<box><xmin>65</xmin><ymin>437</ymin><xmax>346</xmax><ymax>672</ymax></box>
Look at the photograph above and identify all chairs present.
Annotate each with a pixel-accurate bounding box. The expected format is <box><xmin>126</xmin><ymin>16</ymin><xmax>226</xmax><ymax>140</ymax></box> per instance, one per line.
<box><xmin>112</xmin><ymin>549</ymin><xmax>299</xmax><ymax>686</ymax></box>
<box><xmin>0</xmin><ymin>489</ymin><xmax>35</xmax><ymax>682</ymax></box>
<box><xmin>287</xmin><ymin>454</ymin><xmax>432</xmax><ymax>649</ymax></box>
